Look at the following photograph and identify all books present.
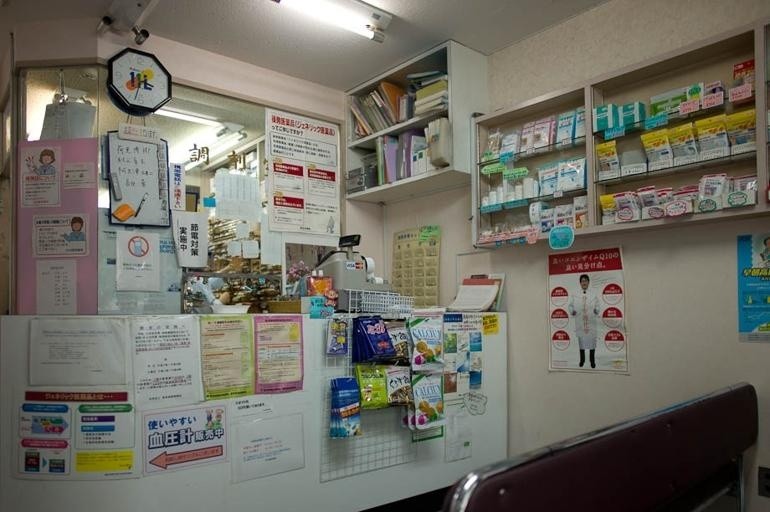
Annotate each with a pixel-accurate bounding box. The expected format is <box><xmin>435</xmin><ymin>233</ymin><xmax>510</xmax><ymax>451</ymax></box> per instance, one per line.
<box><xmin>346</xmin><ymin>69</ymin><xmax>451</xmax><ymax>194</ymax></box>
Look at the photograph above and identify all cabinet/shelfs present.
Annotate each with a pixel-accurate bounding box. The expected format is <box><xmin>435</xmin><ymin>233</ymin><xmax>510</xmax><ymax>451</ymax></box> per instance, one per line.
<box><xmin>346</xmin><ymin>39</ymin><xmax>490</xmax><ymax>205</ymax></box>
<box><xmin>470</xmin><ymin>83</ymin><xmax>590</xmax><ymax>249</ymax></box>
<box><xmin>591</xmin><ymin>22</ymin><xmax>764</xmax><ymax>233</ymax></box>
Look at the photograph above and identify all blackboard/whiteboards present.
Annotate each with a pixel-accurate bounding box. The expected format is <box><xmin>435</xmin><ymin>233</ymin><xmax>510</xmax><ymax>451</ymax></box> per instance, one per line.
<box><xmin>107</xmin><ymin>130</ymin><xmax>171</xmax><ymax>228</ymax></box>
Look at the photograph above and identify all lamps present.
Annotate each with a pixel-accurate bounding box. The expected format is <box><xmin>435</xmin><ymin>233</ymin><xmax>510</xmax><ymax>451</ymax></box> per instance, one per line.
<box><xmin>273</xmin><ymin>0</ymin><xmax>394</xmax><ymax>43</ymax></box>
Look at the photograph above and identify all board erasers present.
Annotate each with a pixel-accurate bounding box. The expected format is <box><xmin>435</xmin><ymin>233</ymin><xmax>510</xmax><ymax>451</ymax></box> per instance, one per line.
<box><xmin>109</xmin><ymin>172</ymin><xmax>122</xmax><ymax>200</ymax></box>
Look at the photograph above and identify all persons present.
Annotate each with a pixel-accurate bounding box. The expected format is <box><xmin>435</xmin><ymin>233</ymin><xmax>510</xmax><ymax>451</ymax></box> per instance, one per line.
<box><xmin>568</xmin><ymin>274</ymin><xmax>600</xmax><ymax>368</ymax></box>
<box><xmin>760</xmin><ymin>236</ymin><xmax>770</xmax><ymax>266</ymax></box>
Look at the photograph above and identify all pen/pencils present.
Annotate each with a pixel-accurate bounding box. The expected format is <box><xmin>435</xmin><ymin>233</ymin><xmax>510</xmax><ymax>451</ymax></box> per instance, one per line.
<box><xmin>134</xmin><ymin>192</ymin><xmax>148</xmax><ymax>218</ymax></box>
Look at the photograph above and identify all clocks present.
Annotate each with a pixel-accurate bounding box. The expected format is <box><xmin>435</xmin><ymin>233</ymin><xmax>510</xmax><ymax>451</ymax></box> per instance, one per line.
<box><xmin>107</xmin><ymin>47</ymin><xmax>173</xmax><ymax>114</ymax></box>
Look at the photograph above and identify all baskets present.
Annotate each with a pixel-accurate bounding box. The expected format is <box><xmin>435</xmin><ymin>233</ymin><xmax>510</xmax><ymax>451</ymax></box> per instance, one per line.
<box><xmin>268</xmin><ymin>300</ymin><xmax>301</xmax><ymax>313</ymax></box>
<box><xmin>361</xmin><ymin>292</ymin><xmax>415</xmax><ymax>314</ymax></box>
